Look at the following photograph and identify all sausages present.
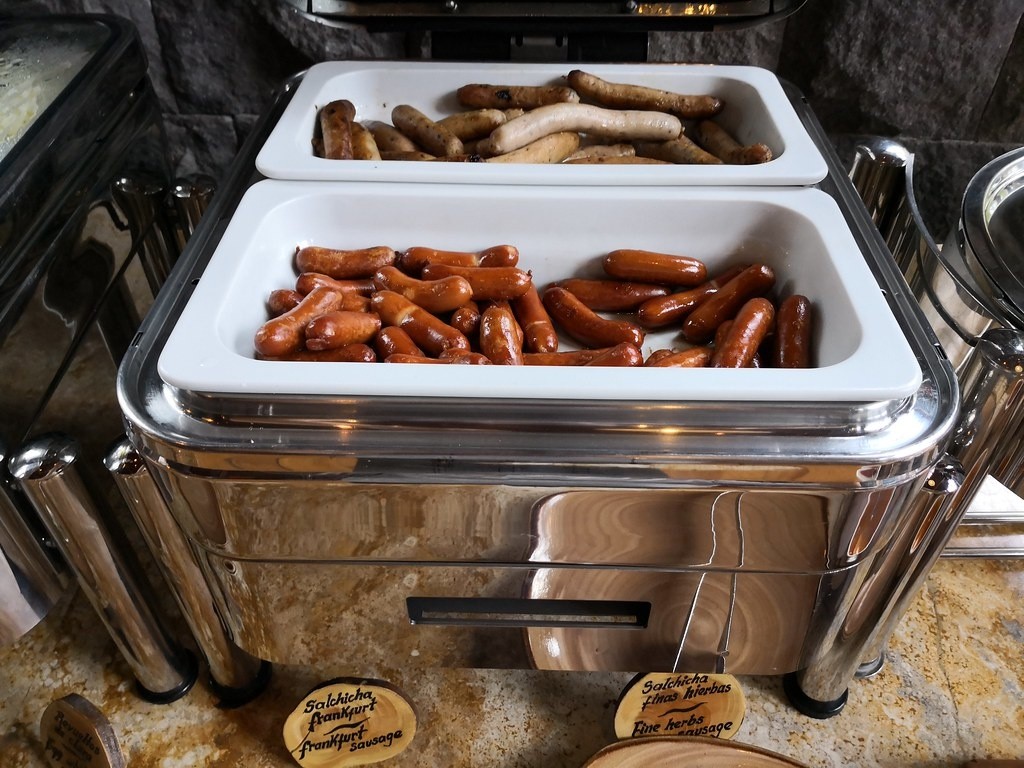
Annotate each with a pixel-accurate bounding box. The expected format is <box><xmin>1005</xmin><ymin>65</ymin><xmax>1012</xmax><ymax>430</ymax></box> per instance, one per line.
<box><xmin>311</xmin><ymin>69</ymin><xmax>774</xmax><ymax>165</ymax></box>
<box><xmin>254</xmin><ymin>244</ymin><xmax>813</xmax><ymax>369</ymax></box>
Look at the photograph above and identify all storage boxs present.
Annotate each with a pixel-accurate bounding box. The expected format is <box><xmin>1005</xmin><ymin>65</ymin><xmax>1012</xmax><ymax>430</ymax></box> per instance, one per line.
<box><xmin>157</xmin><ymin>61</ymin><xmax>923</xmax><ymax>406</ymax></box>
